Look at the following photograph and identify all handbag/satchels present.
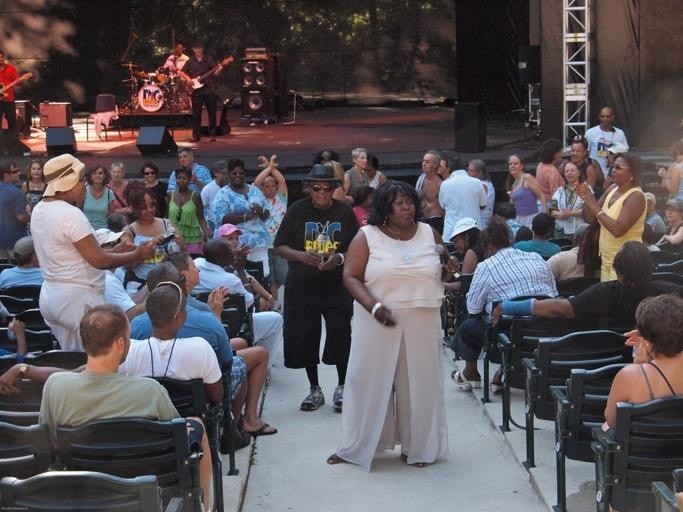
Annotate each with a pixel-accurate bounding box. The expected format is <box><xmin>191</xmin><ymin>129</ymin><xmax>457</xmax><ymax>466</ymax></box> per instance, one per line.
<box><xmin>578</xmin><ymin>218</ymin><xmax>601</xmax><ymax>265</ymax></box>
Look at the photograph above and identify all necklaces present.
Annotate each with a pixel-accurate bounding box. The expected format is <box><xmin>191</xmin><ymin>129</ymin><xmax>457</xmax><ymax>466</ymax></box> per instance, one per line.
<box><xmin>30</xmin><ymin>181</ymin><xmax>42</xmax><ymax>191</ymax></box>
<box><xmin>511</xmin><ymin>175</ymin><xmax>522</xmax><ymax>188</ymax></box>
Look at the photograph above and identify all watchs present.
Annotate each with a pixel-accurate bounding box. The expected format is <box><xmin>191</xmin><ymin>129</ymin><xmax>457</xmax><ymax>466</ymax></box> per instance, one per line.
<box><xmin>18</xmin><ymin>363</ymin><xmax>29</xmax><ymax>378</ymax></box>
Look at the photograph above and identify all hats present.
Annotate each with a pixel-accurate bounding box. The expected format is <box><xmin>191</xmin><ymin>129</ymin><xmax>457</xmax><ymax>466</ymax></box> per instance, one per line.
<box><xmin>665</xmin><ymin>197</ymin><xmax>683</xmax><ymax>211</ymax></box>
<box><xmin>41</xmin><ymin>154</ymin><xmax>86</xmax><ymax>197</ymax></box>
<box><xmin>92</xmin><ymin>226</ymin><xmax>127</xmax><ymax>247</ymax></box>
<box><xmin>218</xmin><ymin>222</ymin><xmax>243</xmax><ymax>239</ymax></box>
<box><xmin>13</xmin><ymin>235</ymin><xmax>34</xmax><ymax>255</ymax></box>
<box><xmin>644</xmin><ymin>192</ymin><xmax>657</xmax><ymax>206</ymax></box>
<box><xmin>304</xmin><ymin>164</ymin><xmax>341</xmax><ymax>182</ymax></box>
<box><xmin>449</xmin><ymin>217</ymin><xmax>482</xmax><ymax>242</ymax></box>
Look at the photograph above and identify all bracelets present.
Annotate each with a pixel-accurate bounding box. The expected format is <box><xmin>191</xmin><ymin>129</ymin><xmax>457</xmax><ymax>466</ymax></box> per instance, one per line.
<box><xmin>268</xmin><ymin>294</ymin><xmax>274</xmax><ymax>301</ymax></box>
<box><xmin>663</xmin><ymin>176</ymin><xmax>671</xmax><ymax>180</ymax></box>
<box><xmin>594</xmin><ymin>208</ymin><xmax>607</xmax><ymax>220</ymax></box>
<box><xmin>261</xmin><ymin>203</ymin><xmax>266</xmax><ymax>220</ymax></box>
<box><xmin>580</xmin><ymin>205</ymin><xmax>588</xmax><ymax>210</ymax></box>
<box><xmin>370</xmin><ymin>303</ymin><xmax>383</xmax><ymax>317</ymax></box>
<box><xmin>242</xmin><ymin>212</ymin><xmax>249</xmax><ymax>223</ymax></box>
<box><xmin>337</xmin><ymin>250</ymin><xmax>345</xmax><ymax>267</ymax></box>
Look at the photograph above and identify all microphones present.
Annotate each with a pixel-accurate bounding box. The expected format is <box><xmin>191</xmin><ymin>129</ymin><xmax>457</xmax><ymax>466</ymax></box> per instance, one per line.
<box><xmin>128</xmin><ymin>60</ymin><xmax>133</xmax><ymax>71</ymax></box>
<box><xmin>436</xmin><ymin>244</ymin><xmax>452</xmax><ymax>262</ymax></box>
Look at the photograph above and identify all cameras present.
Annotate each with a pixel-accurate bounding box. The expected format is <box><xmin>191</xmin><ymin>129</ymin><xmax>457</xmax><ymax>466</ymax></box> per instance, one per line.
<box><xmin>158</xmin><ymin>234</ymin><xmax>175</xmax><ymax>245</ymax></box>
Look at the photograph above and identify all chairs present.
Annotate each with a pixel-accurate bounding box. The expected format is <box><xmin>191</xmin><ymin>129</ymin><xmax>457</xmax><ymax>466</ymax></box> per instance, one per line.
<box><xmin>0</xmin><ymin>285</ymin><xmax>87</xmax><ymax>369</ymax></box>
<box><xmin>212</xmin><ymin>346</ymin><xmax>239</xmax><ymax>475</ymax></box>
<box><xmin>556</xmin><ymin>277</ymin><xmax>600</xmax><ymax>298</ymax></box>
<box><xmin>0</xmin><ymin>421</ymin><xmax>55</xmax><ymax>479</ymax></box>
<box><xmin>650</xmin><ymin>245</ymin><xmax>683</xmax><ymax>285</ymax></box>
<box><xmin>151</xmin><ymin>376</ymin><xmax>223</xmax><ymax>512</ymax></box>
<box><xmin>498</xmin><ymin>315</ymin><xmax>636</xmax><ymax>432</ymax></box>
<box><xmin>453</xmin><ymin>274</ymin><xmax>473</xmax><ymax>361</ymax></box>
<box><xmin>651</xmin><ymin>469</ymin><xmax>683</xmax><ymax>512</ymax></box>
<box><xmin>245</xmin><ymin>259</ymin><xmax>271</xmax><ymax>295</ymax></box>
<box><xmin>590</xmin><ymin>395</ymin><xmax>683</xmax><ymax>512</ymax></box>
<box><xmin>520</xmin><ymin>330</ymin><xmax>633</xmax><ymax>469</ymax></box>
<box><xmin>0</xmin><ymin>379</ymin><xmax>43</xmax><ymax>424</ymax></box>
<box><xmin>196</xmin><ymin>293</ymin><xmax>260</xmax><ymax>348</ymax></box>
<box><xmin>481</xmin><ymin>295</ymin><xmax>553</xmax><ymax>404</ymax></box>
<box><xmin>0</xmin><ymin>471</ymin><xmax>188</xmax><ymax>512</ymax></box>
<box><xmin>57</xmin><ymin>418</ymin><xmax>204</xmax><ymax>512</ymax></box>
<box><xmin>549</xmin><ymin>363</ymin><xmax>628</xmax><ymax>511</ymax></box>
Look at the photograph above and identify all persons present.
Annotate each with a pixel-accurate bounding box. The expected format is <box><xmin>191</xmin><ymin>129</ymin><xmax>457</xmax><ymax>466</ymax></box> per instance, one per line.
<box><xmin>118</xmin><ymin>181</ymin><xmax>179</xmax><ymax>291</ymax></box>
<box><xmin>494</xmin><ymin>201</ymin><xmax>521</xmax><ymax>237</ymax></box>
<box><xmin>466</xmin><ymin>158</ymin><xmax>496</xmax><ymax>229</ymax></box>
<box><xmin>656</xmin><ymin>195</ymin><xmax>683</xmax><ymax>246</ymax></box>
<box><xmin>436</xmin><ymin>153</ymin><xmax>450</xmax><ymax>181</ymax></box>
<box><xmin>179</xmin><ymin>40</ymin><xmax>222</xmax><ymax>142</ymax></box>
<box><xmin>76</xmin><ymin>165</ymin><xmax>115</xmax><ymax>231</ymax></box>
<box><xmin>658</xmin><ymin>142</ymin><xmax>681</xmax><ymax>187</ymax></box>
<box><xmin>253</xmin><ymin>155</ymin><xmax>289</xmax><ymax>316</ymax></box>
<box><xmin>301</xmin><ymin>149</ymin><xmax>340</xmax><ymax>194</ymax></box>
<box><xmin>342</xmin><ymin>148</ymin><xmax>370</xmax><ymax>206</ymax></box>
<box><xmin>137</xmin><ymin>161</ymin><xmax>169</xmax><ymax>216</ymax></box>
<box><xmin>324</xmin><ymin>161</ymin><xmax>354</xmax><ymax>204</ymax></box>
<box><xmin>167</xmin><ymin>146</ymin><xmax>212</xmax><ymax>195</ymax></box>
<box><xmin>505</xmin><ymin>153</ymin><xmax>549</xmax><ymax>225</ymax></box>
<box><xmin>604</xmin><ymin>294</ymin><xmax>683</xmax><ymax>429</ymax></box>
<box><xmin>131</xmin><ymin>264</ymin><xmax>252</xmax><ymax>454</ymax></box>
<box><xmin>0</xmin><ymin>162</ymin><xmax>33</xmax><ymax>260</ymax></box>
<box><xmin>0</xmin><ymin>51</ymin><xmax>22</xmax><ymax>143</ymax></box>
<box><xmin>165</xmin><ymin>45</ymin><xmax>190</xmax><ymax>71</ymax></box>
<box><xmin>574</xmin><ymin>152</ymin><xmax>647</xmax><ymax>282</ymax></box>
<box><xmin>95</xmin><ymin>228</ymin><xmax>149</xmax><ymax>320</ymax></box>
<box><xmin>641</xmin><ymin>223</ymin><xmax>662</xmax><ymax>252</ymax></box>
<box><xmin>272</xmin><ymin>165</ymin><xmax>360</xmax><ymax>412</ymax></box>
<box><xmin>21</xmin><ymin>157</ymin><xmax>48</xmax><ymax>210</ymax></box>
<box><xmin>30</xmin><ymin>153</ymin><xmax>156</xmax><ymax>351</ymax></box>
<box><xmin>200</xmin><ymin>160</ymin><xmax>228</xmax><ymax>226</ymax></box>
<box><xmin>107</xmin><ymin>160</ymin><xmax>135</xmax><ymax>214</ymax></box>
<box><xmin>439</xmin><ymin>152</ymin><xmax>488</xmax><ymax>243</ymax></box>
<box><xmin>441</xmin><ymin>216</ymin><xmax>488</xmax><ymax>347</ymax></box>
<box><xmin>163</xmin><ymin>167</ymin><xmax>211</xmax><ymax>257</ymax></box>
<box><xmin>492</xmin><ymin>241</ymin><xmax>683</xmax><ymax>328</ymax></box>
<box><xmin>349</xmin><ymin>185</ymin><xmax>377</xmax><ymax>229</ymax></box>
<box><xmin>584</xmin><ymin>106</ymin><xmax>629</xmax><ymax>177</ymax></box>
<box><xmin>328</xmin><ymin>181</ymin><xmax>448</xmax><ymax>467</ymax></box>
<box><xmin>514</xmin><ymin>225</ymin><xmax>534</xmax><ymax>244</ymax></box>
<box><xmin>535</xmin><ymin>138</ymin><xmax>565</xmax><ymax>199</ymax></box>
<box><xmin>362</xmin><ymin>154</ymin><xmax>390</xmax><ymax>188</ymax></box>
<box><xmin>564</xmin><ymin>136</ymin><xmax>598</xmax><ymax>199</ymax></box>
<box><xmin>583</xmin><ymin>137</ymin><xmax>605</xmax><ymax>184</ymax></box>
<box><xmin>450</xmin><ymin>216</ymin><xmax>558</xmax><ymax>393</ymax></box>
<box><xmin>513</xmin><ymin>213</ymin><xmax>562</xmax><ymax>261</ymax></box>
<box><xmin>192</xmin><ymin>241</ymin><xmax>256</xmax><ymax>312</ymax></box>
<box><xmin>159</xmin><ymin>251</ymin><xmax>278</xmax><ymax>434</ymax></box>
<box><xmin>414</xmin><ymin>150</ymin><xmax>446</xmax><ymax>234</ymax></box>
<box><xmin>212</xmin><ymin>159</ymin><xmax>270</xmax><ymax>279</ymax></box>
<box><xmin>103</xmin><ymin>212</ymin><xmax>128</xmax><ymax>237</ymax></box>
<box><xmin>0</xmin><ymin>234</ymin><xmax>44</xmax><ymax>287</ymax></box>
<box><xmin>214</xmin><ymin>221</ymin><xmax>274</xmax><ymax>312</ymax></box>
<box><xmin>38</xmin><ymin>303</ymin><xmax>211</xmax><ymax>512</ymax></box>
<box><xmin>547</xmin><ymin>162</ymin><xmax>594</xmax><ymax>240</ymax></box>
<box><xmin>1</xmin><ymin>282</ymin><xmax>224</xmax><ymax>406</ymax></box>
<box><xmin>545</xmin><ymin>223</ymin><xmax>601</xmax><ymax>280</ymax></box>
<box><xmin>662</xmin><ymin>140</ymin><xmax>683</xmax><ymax>199</ymax></box>
<box><xmin>645</xmin><ymin>191</ymin><xmax>667</xmax><ymax>235</ymax></box>
<box><xmin>0</xmin><ymin>317</ymin><xmax>28</xmax><ymax>359</ymax></box>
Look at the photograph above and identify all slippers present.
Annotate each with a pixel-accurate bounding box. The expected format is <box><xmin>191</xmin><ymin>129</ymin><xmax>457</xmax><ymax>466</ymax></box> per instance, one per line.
<box><xmin>241</xmin><ymin>422</ymin><xmax>279</xmax><ymax>436</ymax></box>
<box><xmin>489</xmin><ymin>380</ymin><xmax>504</xmax><ymax>393</ymax></box>
<box><xmin>450</xmin><ymin>368</ymin><xmax>482</xmax><ymax>389</ymax></box>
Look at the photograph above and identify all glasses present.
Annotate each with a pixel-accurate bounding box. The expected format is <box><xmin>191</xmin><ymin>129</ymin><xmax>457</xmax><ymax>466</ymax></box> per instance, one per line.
<box><xmin>156</xmin><ymin>278</ymin><xmax>183</xmax><ymax>318</ymax></box>
<box><xmin>232</xmin><ymin>172</ymin><xmax>244</xmax><ymax>176</ymax></box>
<box><xmin>310</xmin><ymin>184</ymin><xmax>332</xmax><ymax>192</ymax></box>
<box><xmin>105</xmin><ymin>237</ymin><xmax>123</xmax><ymax>246</ymax></box>
<box><xmin>8</xmin><ymin>168</ymin><xmax>19</xmax><ymax>174</ymax></box>
<box><xmin>141</xmin><ymin>201</ymin><xmax>155</xmax><ymax>210</ymax></box>
<box><xmin>611</xmin><ymin>162</ymin><xmax>630</xmax><ymax>170</ymax></box>
<box><xmin>143</xmin><ymin>171</ymin><xmax>156</xmax><ymax>175</ymax></box>
<box><xmin>175</xmin><ymin>207</ymin><xmax>183</xmax><ymax>222</ymax></box>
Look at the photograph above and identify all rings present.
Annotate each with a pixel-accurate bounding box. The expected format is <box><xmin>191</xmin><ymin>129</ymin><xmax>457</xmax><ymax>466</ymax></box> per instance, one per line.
<box><xmin>383</xmin><ymin>320</ymin><xmax>386</xmax><ymax>324</ymax></box>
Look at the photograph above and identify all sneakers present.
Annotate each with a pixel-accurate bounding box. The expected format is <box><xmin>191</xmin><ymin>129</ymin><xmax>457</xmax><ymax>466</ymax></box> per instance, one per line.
<box><xmin>190</xmin><ymin>138</ymin><xmax>201</xmax><ymax>143</ymax></box>
<box><xmin>333</xmin><ymin>385</ymin><xmax>345</xmax><ymax>410</ymax></box>
<box><xmin>209</xmin><ymin>135</ymin><xmax>216</xmax><ymax>143</ymax></box>
<box><xmin>300</xmin><ymin>386</ymin><xmax>325</xmax><ymax>411</ymax></box>
<box><xmin>219</xmin><ymin>433</ymin><xmax>251</xmax><ymax>454</ymax></box>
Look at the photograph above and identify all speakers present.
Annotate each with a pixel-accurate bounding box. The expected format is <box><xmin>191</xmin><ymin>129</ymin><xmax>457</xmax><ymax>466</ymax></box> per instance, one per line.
<box><xmin>238</xmin><ymin>58</ymin><xmax>278</xmax><ymax>123</ymax></box>
<box><xmin>16</xmin><ymin>100</ymin><xmax>33</xmax><ymax>128</ymax></box>
<box><xmin>454</xmin><ymin>101</ymin><xmax>487</xmax><ymax>153</ymax></box>
